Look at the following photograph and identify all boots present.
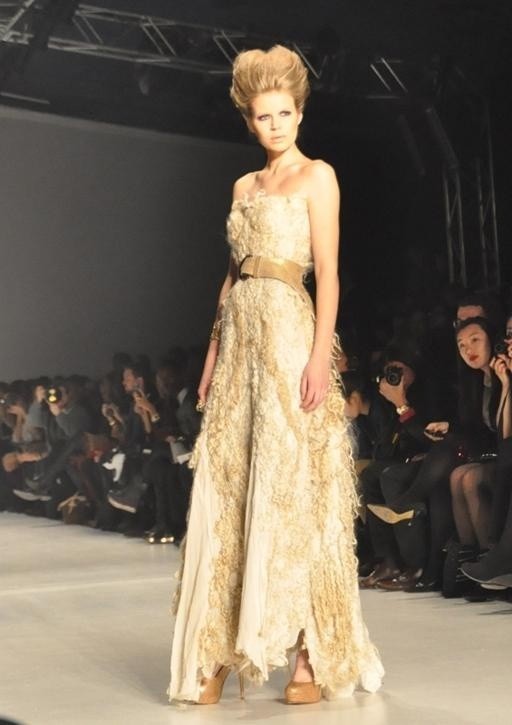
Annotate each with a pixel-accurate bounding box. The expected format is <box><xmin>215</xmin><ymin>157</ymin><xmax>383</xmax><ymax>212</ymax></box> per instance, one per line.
<box><xmin>442</xmin><ymin>541</ymin><xmax>512</xmax><ymax>602</ymax></box>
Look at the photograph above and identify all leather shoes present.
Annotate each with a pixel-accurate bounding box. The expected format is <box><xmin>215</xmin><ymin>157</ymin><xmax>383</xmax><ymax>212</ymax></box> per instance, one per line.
<box><xmin>359</xmin><ymin>567</ymin><xmax>442</xmax><ymax>593</ymax></box>
<box><xmin>13</xmin><ymin>485</ymin><xmax>53</xmax><ymax>502</ymax></box>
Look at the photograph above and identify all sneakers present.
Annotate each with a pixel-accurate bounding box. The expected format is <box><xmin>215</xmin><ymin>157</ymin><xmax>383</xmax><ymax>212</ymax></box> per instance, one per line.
<box><xmin>106</xmin><ymin>492</ymin><xmax>137</xmax><ymax>514</ymax></box>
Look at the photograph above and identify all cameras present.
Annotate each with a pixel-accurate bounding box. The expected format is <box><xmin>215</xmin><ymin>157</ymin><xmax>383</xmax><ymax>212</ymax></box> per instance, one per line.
<box><xmin>489</xmin><ymin>336</ymin><xmax>512</xmax><ymax>358</ymax></box>
<box><xmin>376</xmin><ymin>365</ymin><xmax>404</xmax><ymax>385</ymax></box>
<box><xmin>46</xmin><ymin>387</ymin><xmax>62</xmax><ymax>403</ymax></box>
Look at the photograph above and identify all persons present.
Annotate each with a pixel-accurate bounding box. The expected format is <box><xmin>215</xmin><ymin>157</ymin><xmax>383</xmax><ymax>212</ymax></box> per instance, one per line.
<box><xmin>157</xmin><ymin>40</ymin><xmax>385</xmax><ymax>714</ymax></box>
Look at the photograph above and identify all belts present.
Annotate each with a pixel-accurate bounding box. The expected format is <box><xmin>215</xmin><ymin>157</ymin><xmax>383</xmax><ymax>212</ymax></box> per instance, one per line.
<box><xmin>239</xmin><ymin>255</ymin><xmax>314</xmax><ymax>307</ymax></box>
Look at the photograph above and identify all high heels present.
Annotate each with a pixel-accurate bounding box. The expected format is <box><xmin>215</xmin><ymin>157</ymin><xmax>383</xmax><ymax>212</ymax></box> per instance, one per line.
<box><xmin>368</xmin><ymin>503</ymin><xmax>428</xmax><ymax>526</ymax></box>
<box><xmin>286</xmin><ymin>675</ymin><xmax>320</xmax><ymax>703</ymax></box>
<box><xmin>194</xmin><ymin>665</ymin><xmax>246</xmax><ymax>706</ymax></box>
